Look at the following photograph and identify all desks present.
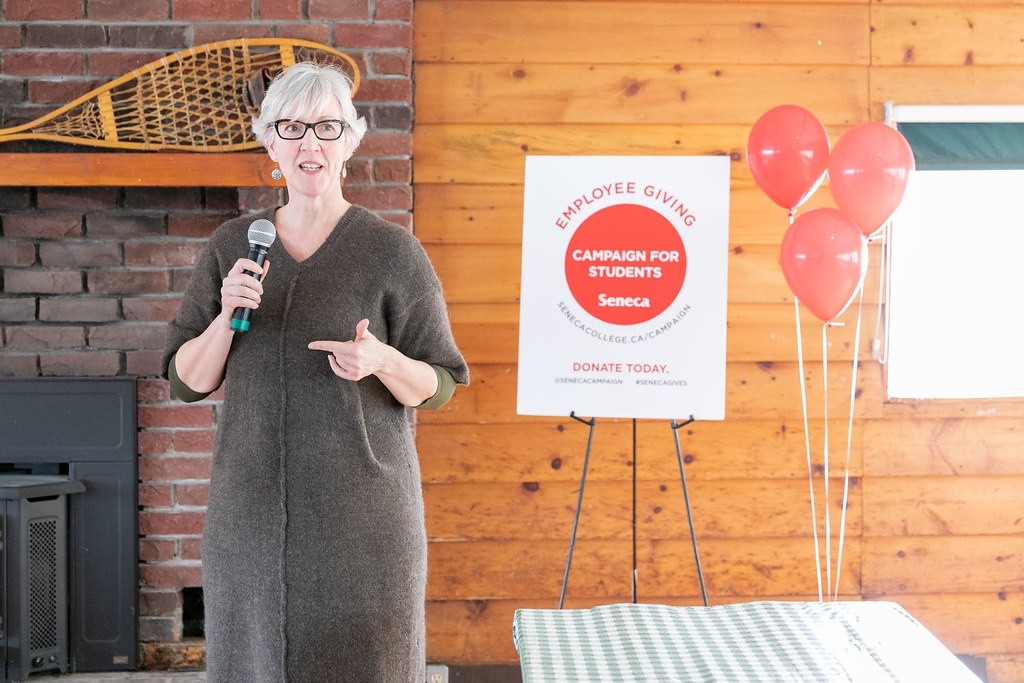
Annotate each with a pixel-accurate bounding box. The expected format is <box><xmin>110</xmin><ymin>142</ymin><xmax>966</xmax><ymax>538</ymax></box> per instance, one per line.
<box><xmin>511</xmin><ymin>600</ymin><xmax>983</xmax><ymax>682</ymax></box>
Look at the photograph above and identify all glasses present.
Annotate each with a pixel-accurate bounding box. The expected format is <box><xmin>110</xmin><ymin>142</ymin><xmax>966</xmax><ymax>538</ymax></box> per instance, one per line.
<box><xmin>267</xmin><ymin>119</ymin><xmax>350</xmax><ymax>141</ymax></box>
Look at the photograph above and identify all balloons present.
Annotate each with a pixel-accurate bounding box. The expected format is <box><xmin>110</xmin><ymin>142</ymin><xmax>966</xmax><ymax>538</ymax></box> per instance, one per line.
<box><xmin>781</xmin><ymin>207</ymin><xmax>869</xmax><ymax>326</ymax></box>
<box><xmin>828</xmin><ymin>120</ymin><xmax>916</xmax><ymax>242</ymax></box>
<box><xmin>746</xmin><ymin>105</ymin><xmax>831</xmax><ymax>218</ymax></box>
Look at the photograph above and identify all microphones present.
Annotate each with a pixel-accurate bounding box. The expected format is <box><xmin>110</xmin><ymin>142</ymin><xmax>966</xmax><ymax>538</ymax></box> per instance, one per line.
<box><xmin>230</xmin><ymin>219</ymin><xmax>277</xmax><ymax>333</ymax></box>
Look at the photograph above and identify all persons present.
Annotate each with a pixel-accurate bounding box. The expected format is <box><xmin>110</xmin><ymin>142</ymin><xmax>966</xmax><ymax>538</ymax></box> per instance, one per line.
<box><xmin>159</xmin><ymin>60</ymin><xmax>471</xmax><ymax>682</ymax></box>
